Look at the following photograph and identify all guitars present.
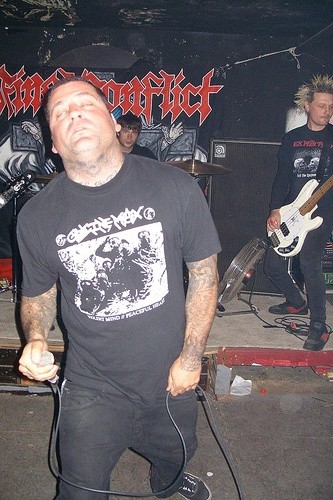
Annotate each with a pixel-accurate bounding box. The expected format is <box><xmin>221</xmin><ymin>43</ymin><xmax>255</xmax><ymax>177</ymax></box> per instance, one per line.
<box><xmin>266</xmin><ymin>174</ymin><xmax>333</xmax><ymax>258</ymax></box>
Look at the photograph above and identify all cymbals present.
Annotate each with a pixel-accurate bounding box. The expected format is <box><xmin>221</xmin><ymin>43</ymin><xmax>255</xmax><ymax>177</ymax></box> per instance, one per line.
<box><xmin>165</xmin><ymin>156</ymin><xmax>232</xmax><ymax>177</ymax></box>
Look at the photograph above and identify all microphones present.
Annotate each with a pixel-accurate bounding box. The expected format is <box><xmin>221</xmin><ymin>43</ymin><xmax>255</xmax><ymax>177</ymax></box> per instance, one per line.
<box><xmin>38</xmin><ymin>351</ymin><xmax>58</xmax><ymax>386</ymax></box>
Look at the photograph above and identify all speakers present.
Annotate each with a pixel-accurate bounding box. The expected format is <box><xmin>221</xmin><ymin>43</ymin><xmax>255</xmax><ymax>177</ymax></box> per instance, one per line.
<box><xmin>208</xmin><ymin>139</ymin><xmax>292</xmax><ymax>297</ymax></box>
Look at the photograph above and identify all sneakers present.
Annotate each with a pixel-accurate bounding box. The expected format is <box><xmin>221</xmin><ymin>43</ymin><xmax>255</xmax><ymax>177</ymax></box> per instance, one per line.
<box><xmin>269</xmin><ymin>302</ymin><xmax>308</xmax><ymax>314</ymax></box>
<box><xmin>303</xmin><ymin>320</ymin><xmax>329</xmax><ymax>351</ymax></box>
<box><xmin>177</xmin><ymin>472</ymin><xmax>211</xmax><ymax>500</ymax></box>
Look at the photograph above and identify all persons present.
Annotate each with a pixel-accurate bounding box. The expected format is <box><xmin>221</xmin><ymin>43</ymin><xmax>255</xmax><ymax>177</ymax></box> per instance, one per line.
<box><xmin>114</xmin><ymin>113</ymin><xmax>158</xmax><ymax>160</ymax></box>
<box><xmin>14</xmin><ymin>78</ymin><xmax>223</xmax><ymax>500</ymax></box>
<box><xmin>265</xmin><ymin>75</ymin><xmax>333</xmax><ymax>352</ymax></box>
